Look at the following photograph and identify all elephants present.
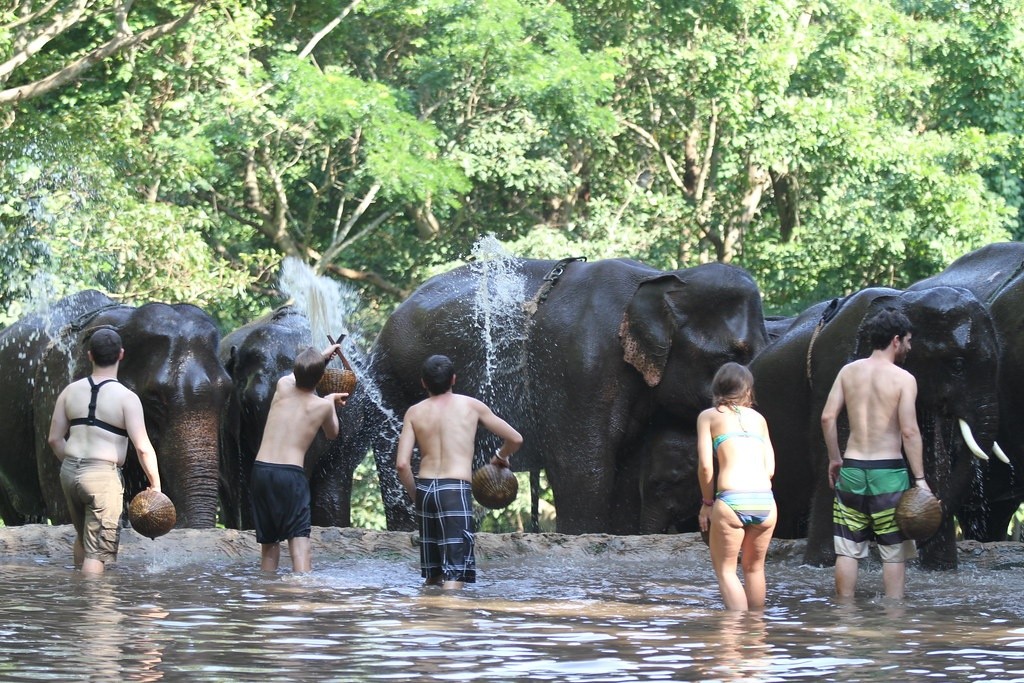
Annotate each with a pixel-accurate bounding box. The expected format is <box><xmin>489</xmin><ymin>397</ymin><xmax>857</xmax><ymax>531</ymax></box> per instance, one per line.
<box><xmin>351</xmin><ymin>237</ymin><xmax>1023</xmax><ymax>574</ymax></box>
<box><xmin>0</xmin><ymin>288</ymin><xmax>236</xmax><ymax>533</ymax></box>
<box><xmin>211</xmin><ymin>303</ymin><xmax>386</xmax><ymax>532</ymax></box>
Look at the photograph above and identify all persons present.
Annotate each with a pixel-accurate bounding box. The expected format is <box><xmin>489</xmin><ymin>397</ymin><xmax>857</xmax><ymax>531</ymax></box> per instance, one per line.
<box><xmin>396</xmin><ymin>355</ymin><xmax>522</xmax><ymax>589</ymax></box>
<box><xmin>697</xmin><ymin>362</ymin><xmax>778</xmax><ymax>610</ymax></box>
<box><xmin>822</xmin><ymin>312</ymin><xmax>931</xmax><ymax>598</ymax></box>
<box><xmin>48</xmin><ymin>329</ymin><xmax>161</xmax><ymax>572</ymax></box>
<box><xmin>252</xmin><ymin>344</ymin><xmax>349</xmax><ymax>574</ymax></box>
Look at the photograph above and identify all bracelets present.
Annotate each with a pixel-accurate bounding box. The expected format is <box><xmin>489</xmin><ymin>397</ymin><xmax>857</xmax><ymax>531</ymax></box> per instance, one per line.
<box><xmin>495</xmin><ymin>448</ymin><xmax>509</xmax><ymax>462</ymax></box>
<box><xmin>702</xmin><ymin>498</ymin><xmax>715</xmax><ymax>505</ymax></box>
<box><xmin>915</xmin><ymin>477</ymin><xmax>926</xmax><ymax>480</ymax></box>
